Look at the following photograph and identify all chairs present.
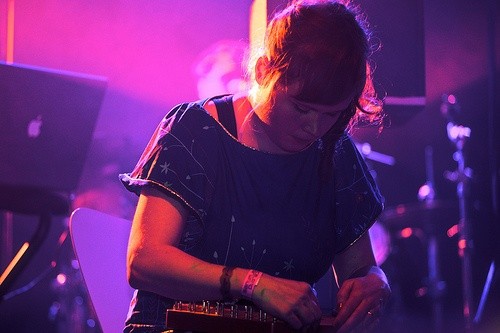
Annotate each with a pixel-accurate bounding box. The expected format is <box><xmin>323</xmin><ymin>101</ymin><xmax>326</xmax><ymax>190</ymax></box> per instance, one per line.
<box><xmin>70</xmin><ymin>206</ymin><xmax>135</xmax><ymax>333</ymax></box>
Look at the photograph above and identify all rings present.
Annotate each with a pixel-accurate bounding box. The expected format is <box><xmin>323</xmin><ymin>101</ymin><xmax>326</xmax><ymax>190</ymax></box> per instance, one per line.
<box><xmin>367</xmin><ymin>311</ymin><xmax>374</xmax><ymax>317</ymax></box>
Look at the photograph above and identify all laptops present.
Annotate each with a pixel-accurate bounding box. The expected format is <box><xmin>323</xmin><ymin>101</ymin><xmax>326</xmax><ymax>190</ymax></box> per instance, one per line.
<box><xmin>0</xmin><ymin>62</ymin><xmax>109</xmax><ymax>193</ymax></box>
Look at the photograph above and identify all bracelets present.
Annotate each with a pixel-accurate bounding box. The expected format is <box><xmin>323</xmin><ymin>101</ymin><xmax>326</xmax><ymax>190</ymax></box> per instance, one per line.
<box><xmin>240</xmin><ymin>269</ymin><xmax>263</xmax><ymax>301</ymax></box>
<box><xmin>219</xmin><ymin>265</ymin><xmax>240</xmax><ymax>294</ymax></box>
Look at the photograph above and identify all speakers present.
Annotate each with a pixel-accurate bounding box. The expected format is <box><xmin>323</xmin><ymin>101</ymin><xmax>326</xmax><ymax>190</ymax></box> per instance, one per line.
<box><xmin>248</xmin><ymin>0</ymin><xmax>427</xmax><ymax>128</ymax></box>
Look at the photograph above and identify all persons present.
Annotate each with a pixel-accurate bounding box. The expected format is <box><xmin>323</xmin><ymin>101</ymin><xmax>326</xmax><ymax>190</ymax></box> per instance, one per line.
<box><xmin>118</xmin><ymin>0</ymin><xmax>390</xmax><ymax>333</ymax></box>
